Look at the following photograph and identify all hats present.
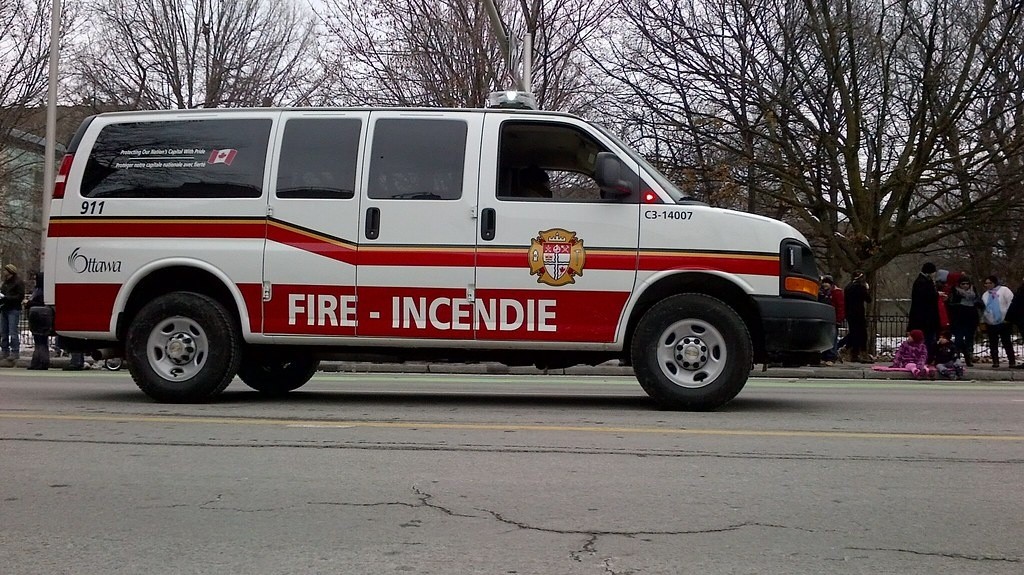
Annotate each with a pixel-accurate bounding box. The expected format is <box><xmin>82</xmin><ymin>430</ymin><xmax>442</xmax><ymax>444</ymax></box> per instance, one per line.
<box><xmin>958</xmin><ymin>275</ymin><xmax>970</xmax><ymax>283</ymax></box>
<box><xmin>940</xmin><ymin>330</ymin><xmax>952</xmax><ymax>339</ymax></box>
<box><xmin>4</xmin><ymin>264</ymin><xmax>18</xmax><ymax>275</ymax></box>
<box><xmin>921</xmin><ymin>262</ymin><xmax>937</xmax><ymax>273</ymax></box>
<box><xmin>821</xmin><ymin>275</ymin><xmax>834</xmax><ymax>284</ymax></box>
<box><xmin>911</xmin><ymin>329</ymin><xmax>924</xmax><ymax>344</ymax></box>
<box><xmin>852</xmin><ymin>270</ymin><xmax>865</xmax><ymax>281</ymax></box>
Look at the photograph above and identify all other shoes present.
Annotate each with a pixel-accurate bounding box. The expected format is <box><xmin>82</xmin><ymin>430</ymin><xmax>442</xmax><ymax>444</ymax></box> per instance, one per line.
<box><xmin>0</xmin><ymin>351</ymin><xmax>10</xmax><ymax>360</ymax></box>
<box><xmin>917</xmin><ymin>368</ymin><xmax>926</xmax><ymax>380</ymax></box>
<box><xmin>928</xmin><ymin>370</ymin><xmax>935</xmax><ymax>381</ymax></box>
<box><xmin>6</xmin><ymin>352</ymin><xmax>19</xmax><ymax>361</ymax></box>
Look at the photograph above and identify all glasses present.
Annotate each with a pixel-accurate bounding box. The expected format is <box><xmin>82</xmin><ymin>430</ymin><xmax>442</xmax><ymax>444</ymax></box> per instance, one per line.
<box><xmin>962</xmin><ymin>283</ymin><xmax>969</xmax><ymax>285</ymax></box>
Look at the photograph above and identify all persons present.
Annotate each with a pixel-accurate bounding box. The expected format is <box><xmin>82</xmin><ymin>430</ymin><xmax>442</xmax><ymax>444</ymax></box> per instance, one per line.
<box><xmin>25</xmin><ymin>272</ymin><xmax>51</xmax><ymax>370</ymax></box>
<box><xmin>0</xmin><ymin>263</ymin><xmax>25</xmax><ymax>359</ymax></box>
<box><xmin>888</xmin><ymin>261</ymin><xmax>1024</xmax><ymax>380</ymax></box>
<box><xmin>53</xmin><ymin>336</ymin><xmax>85</xmax><ymax>370</ymax></box>
<box><xmin>528</xmin><ymin>172</ymin><xmax>552</xmax><ymax>198</ymax></box>
<box><xmin>820</xmin><ymin>267</ymin><xmax>874</xmax><ymax>368</ymax></box>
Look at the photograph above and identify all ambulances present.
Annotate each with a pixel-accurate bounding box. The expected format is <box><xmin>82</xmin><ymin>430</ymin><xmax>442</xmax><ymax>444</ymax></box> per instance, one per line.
<box><xmin>28</xmin><ymin>90</ymin><xmax>838</xmax><ymax>412</ymax></box>
